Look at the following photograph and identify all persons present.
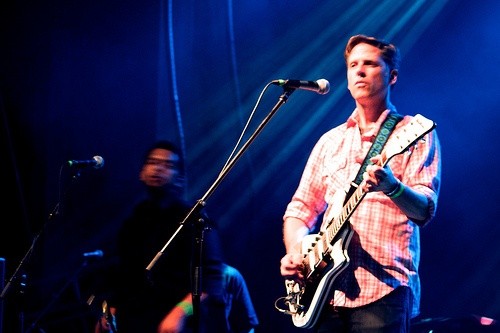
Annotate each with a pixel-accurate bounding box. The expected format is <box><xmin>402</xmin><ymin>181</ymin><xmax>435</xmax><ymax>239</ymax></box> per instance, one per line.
<box><xmin>95</xmin><ymin>142</ymin><xmax>228</xmax><ymax>333</ymax></box>
<box><xmin>281</xmin><ymin>34</ymin><xmax>441</xmax><ymax>333</ymax></box>
<box><xmin>221</xmin><ymin>264</ymin><xmax>259</xmax><ymax>333</ymax></box>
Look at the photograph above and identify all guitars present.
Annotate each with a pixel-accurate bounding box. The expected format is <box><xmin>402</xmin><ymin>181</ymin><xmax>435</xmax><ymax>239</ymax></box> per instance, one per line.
<box><xmin>284</xmin><ymin>113</ymin><xmax>437</xmax><ymax>330</ymax></box>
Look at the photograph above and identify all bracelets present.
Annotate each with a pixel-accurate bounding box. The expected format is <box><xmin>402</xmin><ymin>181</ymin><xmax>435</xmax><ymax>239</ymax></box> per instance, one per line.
<box><xmin>177</xmin><ymin>302</ymin><xmax>193</xmax><ymax>316</ymax></box>
<box><xmin>385</xmin><ymin>178</ymin><xmax>404</xmax><ymax>199</ymax></box>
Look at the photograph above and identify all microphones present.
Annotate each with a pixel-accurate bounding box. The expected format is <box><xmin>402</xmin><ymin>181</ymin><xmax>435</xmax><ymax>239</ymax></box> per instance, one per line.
<box><xmin>270</xmin><ymin>78</ymin><xmax>331</xmax><ymax>95</ymax></box>
<box><xmin>66</xmin><ymin>155</ymin><xmax>104</xmax><ymax>169</ymax></box>
